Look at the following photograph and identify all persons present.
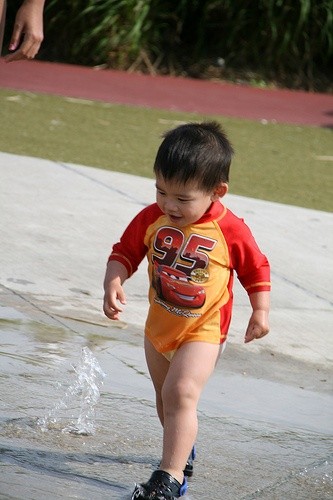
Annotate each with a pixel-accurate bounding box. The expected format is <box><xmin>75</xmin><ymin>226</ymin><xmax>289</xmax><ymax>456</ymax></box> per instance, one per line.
<box><xmin>0</xmin><ymin>0</ymin><xmax>44</xmax><ymax>63</ymax></box>
<box><xmin>103</xmin><ymin>124</ymin><xmax>270</xmax><ymax>500</ymax></box>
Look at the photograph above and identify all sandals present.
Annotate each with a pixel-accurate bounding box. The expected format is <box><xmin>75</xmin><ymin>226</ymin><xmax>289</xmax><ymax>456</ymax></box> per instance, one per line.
<box><xmin>132</xmin><ymin>470</ymin><xmax>187</xmax><ymax>500</ymax></box>
<box><xmin>182</xmin><ymin>445</ymin><xmax>196</xmax><ymax>477</ymax></box>
